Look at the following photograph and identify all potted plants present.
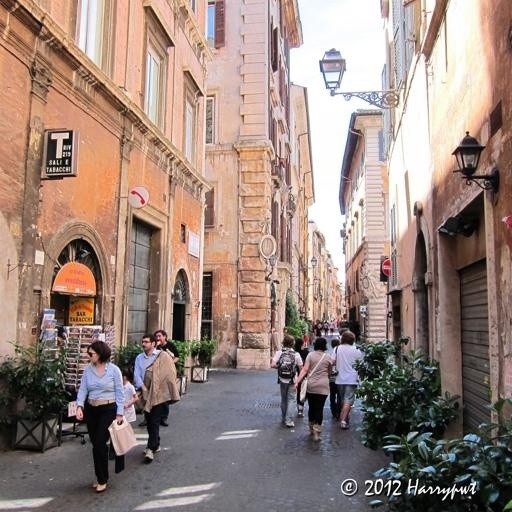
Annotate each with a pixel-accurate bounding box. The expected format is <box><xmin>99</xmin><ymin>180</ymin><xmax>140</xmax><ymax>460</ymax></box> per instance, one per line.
<box><xmin>0</xmin><ymin>328</ymin><xmax>82</xmax><ymax>454</ymax></box>
<box><xmin>110</xmin><ymin>335</ymin><xmax>219</xmax><ymax>413</ymax></box>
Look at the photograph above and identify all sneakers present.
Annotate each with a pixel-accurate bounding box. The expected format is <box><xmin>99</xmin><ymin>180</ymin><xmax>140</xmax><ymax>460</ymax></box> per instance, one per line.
<box><xmin>282</xmin><ymin>418</ymin><xmax>295</xmax><ymax>427</ymax></box>
<box><xmin>296</xmin><ymin>405</ymin><xmax>305</xmax><ymax>417</ymax></box>
<box><xmin>332</xmin><ymin>412</ymin><xmax>351</xmax><ymax>429</ymax></box>
<box><xmin>143</xmin><ymin>444</ymin><xmax>161</xmax><ymax>460</ymax></box>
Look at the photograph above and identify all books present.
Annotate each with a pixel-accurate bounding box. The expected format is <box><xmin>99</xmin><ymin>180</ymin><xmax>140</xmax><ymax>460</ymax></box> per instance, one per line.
<box><xmin>39</xmin><ymin>309</ymin><xmax>105</xmax><ymax>402</ymax></box>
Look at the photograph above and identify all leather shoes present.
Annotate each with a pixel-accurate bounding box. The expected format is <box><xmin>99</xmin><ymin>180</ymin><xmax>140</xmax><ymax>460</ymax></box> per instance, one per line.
<box><xmin>139</xmin><ymin>420</ymin><xmax>147</xmax><ymax>426</ymax></box>
<box><xmin>159</xmin><ymin>420</ymin><xmax>169</xmax><ymax>427</ymax></box>
<box><xmin>92</xmin><ymin>477</ymin><xmax>107</xmax><ymax>492</ymax></box>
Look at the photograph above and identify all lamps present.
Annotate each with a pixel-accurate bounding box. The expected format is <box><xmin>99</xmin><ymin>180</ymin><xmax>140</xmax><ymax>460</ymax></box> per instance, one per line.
<box><xmin>319</xmin><ymin>49</ymin><xmax>399</xmax><ymax>110</ymax></box>
<box><xmin>452</xmin><ymin>133</ymin><xmax>499</xmax><ymax>195</ymax></box>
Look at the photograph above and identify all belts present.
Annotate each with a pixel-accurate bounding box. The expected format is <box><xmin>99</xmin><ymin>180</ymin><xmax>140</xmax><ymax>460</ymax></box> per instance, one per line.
<box><xmin>88</xmin><ymin>398</ymin><xmax>116</xmax><ymax>407</ymax></box>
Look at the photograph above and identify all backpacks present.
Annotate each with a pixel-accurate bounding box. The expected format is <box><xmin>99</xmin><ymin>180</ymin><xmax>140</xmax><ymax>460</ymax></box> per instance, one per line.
<box><xmin>279</xmin><ymin>352</ymin><xmax>295</xmax><ymax>378</ymax></box>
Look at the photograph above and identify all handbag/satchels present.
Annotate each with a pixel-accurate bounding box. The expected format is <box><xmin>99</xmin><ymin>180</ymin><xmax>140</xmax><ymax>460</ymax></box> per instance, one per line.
<box><xmin>299</xmin><ymin>378</ymin><xmax>308</xmax><ymax>403</ymax></box>
<box><xmin>108</xmin><ymin>417</ymin><xmax>138</xmax><ymax>456</ymax></box>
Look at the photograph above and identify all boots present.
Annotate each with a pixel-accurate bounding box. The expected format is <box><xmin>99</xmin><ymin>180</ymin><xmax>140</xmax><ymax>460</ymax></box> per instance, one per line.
<box><xmin>308</xmin><ymin>422</ymin><xmax>322</xmax><ymax>442</ymax></box>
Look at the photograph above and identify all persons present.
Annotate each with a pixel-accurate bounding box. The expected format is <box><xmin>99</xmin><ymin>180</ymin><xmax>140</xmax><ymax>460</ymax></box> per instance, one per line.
<box><xmin>133</xmin><ymin>334</ymin><xmax>182</xmax><ymax>463</ymax></box>
<box><xmin>75</xmin><ymin>338</ymin><xmax>127</xmax><ymax>493</ymax></box>
<box><xmin>121</xmin><ymin>367</ymin><xmax>140</xmax><ymax>424</ymax></box>
<box><xmin>271</xmin><ymin>318</ymin><xmax>366</xmax><ymax>443</ymax></box>
<box><xmin>138</xmin><ymin>330</ymin><xmax>181</xmax><ymax>427</ymax></box>
